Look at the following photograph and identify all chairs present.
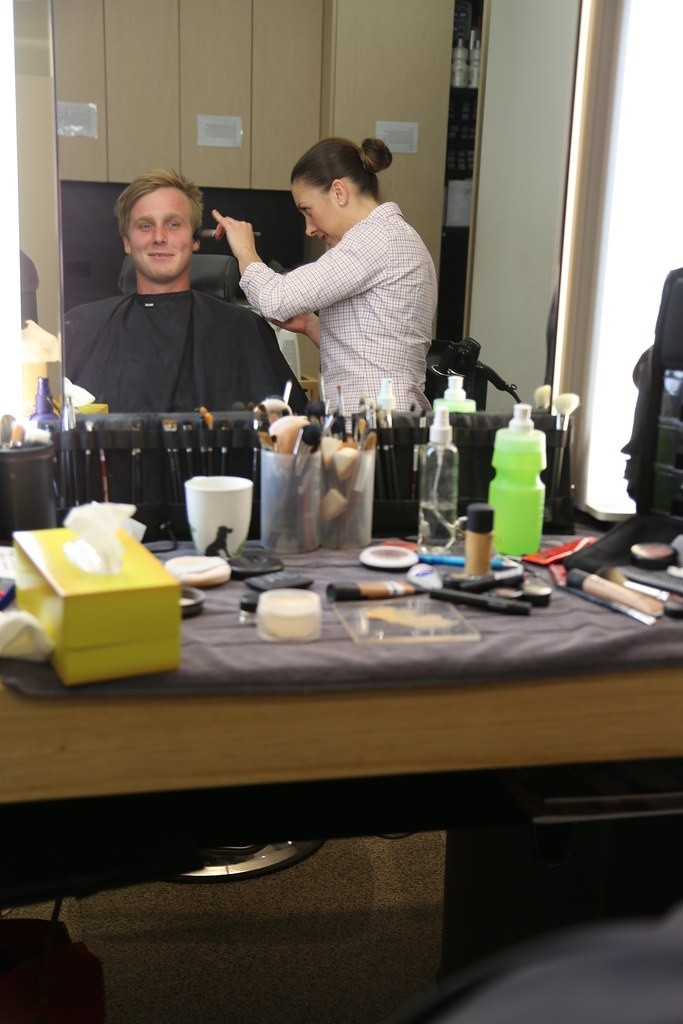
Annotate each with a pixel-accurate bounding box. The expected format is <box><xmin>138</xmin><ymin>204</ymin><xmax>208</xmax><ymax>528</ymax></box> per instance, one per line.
<box><xmin>118</xmin><ymin>253</ymin><xmax>240</xmax><ymax>302</ymax></box>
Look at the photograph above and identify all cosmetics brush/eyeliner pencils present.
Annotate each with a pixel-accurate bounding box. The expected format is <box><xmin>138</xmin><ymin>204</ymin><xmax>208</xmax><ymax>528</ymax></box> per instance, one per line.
<box><xmin>550</xmin><ymin>393</ymin><xmax>580</xmax><ymax>498</ymax></box>
<box><xmin>64</xmin><ymin>383</ymin><xmax>427</xmax><ymax>550</ymax></box>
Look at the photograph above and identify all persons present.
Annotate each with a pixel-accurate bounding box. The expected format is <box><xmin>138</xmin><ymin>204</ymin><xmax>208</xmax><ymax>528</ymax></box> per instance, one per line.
<box><xmin>63</xmin><ymin>168</ymin><xmax>310</xmax><ymax>413</ymax></box>
<box><xmin>212</xmin><ymin>137</ymin><xmax>438</xmax><ymax>433</ymax></box>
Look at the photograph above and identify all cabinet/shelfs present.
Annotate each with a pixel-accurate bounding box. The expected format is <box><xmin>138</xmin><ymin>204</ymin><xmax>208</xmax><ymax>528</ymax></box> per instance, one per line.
<box><xmin>323</xmin><ymin>0</ymin><xmax>483</xmax><ymax>341</ymax></box>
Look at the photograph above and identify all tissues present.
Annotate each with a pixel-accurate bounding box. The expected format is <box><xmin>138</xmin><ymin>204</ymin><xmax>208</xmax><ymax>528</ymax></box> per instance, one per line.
<box><xmin>11</xmin><ymin>499</ymin><xmax>181</xmax><ymax>689</ymax></box>
<box><xmin>64</xmin><ymin>375</ymin><xmax>107</xmax><ymax>415</ymax></box>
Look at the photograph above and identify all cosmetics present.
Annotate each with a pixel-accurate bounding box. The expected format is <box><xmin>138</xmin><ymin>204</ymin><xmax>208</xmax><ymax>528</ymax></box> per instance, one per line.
<box><xmin>552</xmin><ymin>532</ymin><xmax>683</xmax><ymax>628</ymax></box>
<box><xmin>452</xmin><ymin>29</ymin><xmax>482</xmax><ymax>89</ymax></box>
<box><xmin>167</xmin><ymin>504</ymin><xmax>551</xmax><ymax>642</ymax></box>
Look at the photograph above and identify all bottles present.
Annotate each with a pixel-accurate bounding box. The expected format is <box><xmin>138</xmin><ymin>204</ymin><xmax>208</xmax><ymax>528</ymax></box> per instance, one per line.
<box><xmin>487</xmin><ymin>402</ymin><xmax>547</xmax><ymax>557</ymax></box>
<box><xmin>418</xmin><ymin>405</ymin><xmax>459</xmax><ymax>553</ymax></box>
<box><xmin>432</xmin><ymin>375</ymin><xmax>477</xmax><ymax>414</ymax></box>
<box><xmin>464</xmin><ymin>503</ymin><xmax>495</xmax><ymax>579</ymax></box>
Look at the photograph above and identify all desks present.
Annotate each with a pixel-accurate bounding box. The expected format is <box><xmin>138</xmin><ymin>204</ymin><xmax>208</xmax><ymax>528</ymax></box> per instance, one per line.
<box><xmin>0</xmin><ymin>539</ymin><xmax>683</xmax><ymax>974</ymax></box>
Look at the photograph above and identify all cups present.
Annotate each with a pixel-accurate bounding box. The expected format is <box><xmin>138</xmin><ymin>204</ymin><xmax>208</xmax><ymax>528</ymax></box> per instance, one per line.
<box><xmin>184</xmin><ymin>475</ymin><xmax>254</xmax><ymax>561</ymax></box>
<box><xmin>319</xmin><ymin>448</ymin><xmax>377</xmax><ymax>548</ymax></box>
<box><xmin>260</xmin><ymin>448</ymin><xmax>321</xmax><ymax>554</ymax></box>
<box><xmin>0</xmin><ymin>442</ymin><xmax>55</xmax><ymax>547</ymax></box>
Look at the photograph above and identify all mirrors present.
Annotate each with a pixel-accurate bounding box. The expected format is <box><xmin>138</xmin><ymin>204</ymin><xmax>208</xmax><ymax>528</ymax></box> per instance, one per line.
<box><xmin>13</xmin><ymin>0</ymin><xmax>626</xmax><ymax>488</ymax></box>
